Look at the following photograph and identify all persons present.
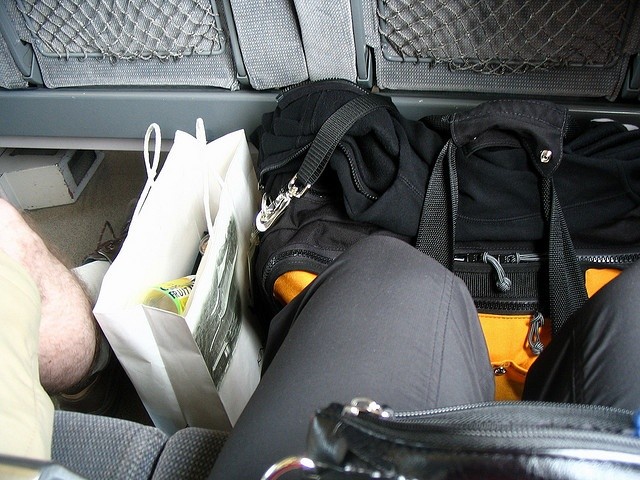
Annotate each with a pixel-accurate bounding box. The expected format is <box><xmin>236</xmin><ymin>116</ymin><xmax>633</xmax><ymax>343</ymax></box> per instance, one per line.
<box><xmin>0</xmin><ymin>197</ymin><xmax>135</xmax><ymax>480</ymax></box>
<box><xmin>209</xmin><ymin>234</ymin><xmax>639</xmax><ymax>480</ymax></box>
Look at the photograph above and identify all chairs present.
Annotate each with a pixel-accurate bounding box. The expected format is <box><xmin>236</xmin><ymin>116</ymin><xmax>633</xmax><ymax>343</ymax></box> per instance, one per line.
<box><xmin>1</xmin><ymin>1</ymin><xmax>307</xmax><ymax>216</ymax></box>
<box><xmin>50</xmin><ymin>408</ymin><xmax>168</xmax><ymax>479</ymax></box>
<box><xmin>294</xmin><ymin>0</ymin><xmax>640</xmax><ymax>138</ymax></box>
<box><xmin>151</xmin><ymin>426</ymin><xmax>231</xmax><ymax>480</ymax></box>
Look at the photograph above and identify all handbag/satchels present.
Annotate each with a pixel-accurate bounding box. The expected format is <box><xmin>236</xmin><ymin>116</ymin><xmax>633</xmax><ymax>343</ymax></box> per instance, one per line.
<box><xmin>92</xmin><ymin>118</ymin><xmax>265</xmax><ymax>436</ymax></box>
<box><xmin>251</xmin><ymin>93</ymin><xmax>639</xmax><ymax>401</ymax></box>
<box><xmin>260</xmin><ymin>398</ymin><xmax>639</xmax><ymax>480</ymax></box>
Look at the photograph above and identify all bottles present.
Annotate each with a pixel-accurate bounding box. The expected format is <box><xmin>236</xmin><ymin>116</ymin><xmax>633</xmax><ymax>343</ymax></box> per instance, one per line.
<box><xmin>144</xmin><ymin>275</ymin><xmax>195</xmax><ymax>317</ymax></box>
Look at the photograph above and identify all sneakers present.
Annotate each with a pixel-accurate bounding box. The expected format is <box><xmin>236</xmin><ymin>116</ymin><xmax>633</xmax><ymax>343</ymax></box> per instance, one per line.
<box><xmin>56</xmin><ymin>197</ymin><xmax>140</xmax><ymax>417</ymax></box>
